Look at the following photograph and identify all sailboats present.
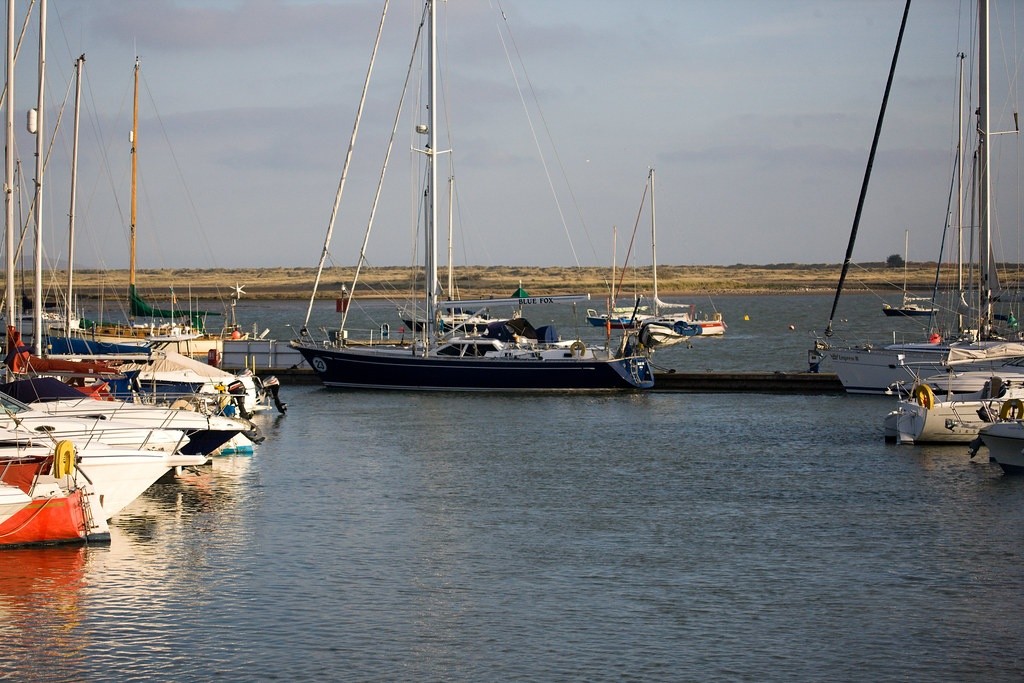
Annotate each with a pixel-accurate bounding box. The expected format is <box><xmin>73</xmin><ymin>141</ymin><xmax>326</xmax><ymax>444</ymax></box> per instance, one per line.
<box><xmin>282</xmin><ymin>1</ymin><xmax>656</xmax><ymax>393</ymax></box>
<box><xmin>396</xmin><ymin>174</ymin><xmax>511</xmax><ymax>332</ymax></box>
<box><xmin>585</xmin><ymin>227</ymin><xmax>654</xmax><ymax>327</ymax></box>
<box><xmin>607</xmin><ymin>169</ymin><xmax>731</xmax><ymax>334</ymax></box>
<box><xmin>809</xmin><ymin>0</ymin><xmax>1024</xmax><ymax>471</ymax></box>
<box><xmin>0</xmin><ymin>1</ymin><xmax>281</xmax><ymax>548</ymax></box>
<box><xmin>876</xmin><ymin>229</ymin><xmax>942</xmax><ymax>318</ymax></box>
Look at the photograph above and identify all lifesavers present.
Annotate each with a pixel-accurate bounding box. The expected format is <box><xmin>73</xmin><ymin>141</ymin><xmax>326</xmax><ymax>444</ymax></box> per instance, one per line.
<box><xmin>570</xmin><ymin>342</ymin><xmax>586</xmax><ymax>356</ymax></box>
<box><xmin>54</xmin><ymin>439</ymin><xmax>74</xmax><ymax>478</ymax></box>
<box><xmin>916</xmin><ymin>384</ymin><xmax>934</xmax><ymax>410</ymax></box>
<box><xmin>705</xmin><ymin>314</ymin><xmax>708</xmax><ymax>322</ymax></box>
<box><xmin>1000</xmin><ymin>398</ymin><xmax>1024</xmax><ymax>422</ymax></box>
<box><xmin>168</xmin><ymin>400</ymin><xmax>193</xmax><ymax>411</ymax></box>
<box><xmin>714</xmin><ymin>313</ymin><xmax>722</xmax><ymax>321</ymax></box>
